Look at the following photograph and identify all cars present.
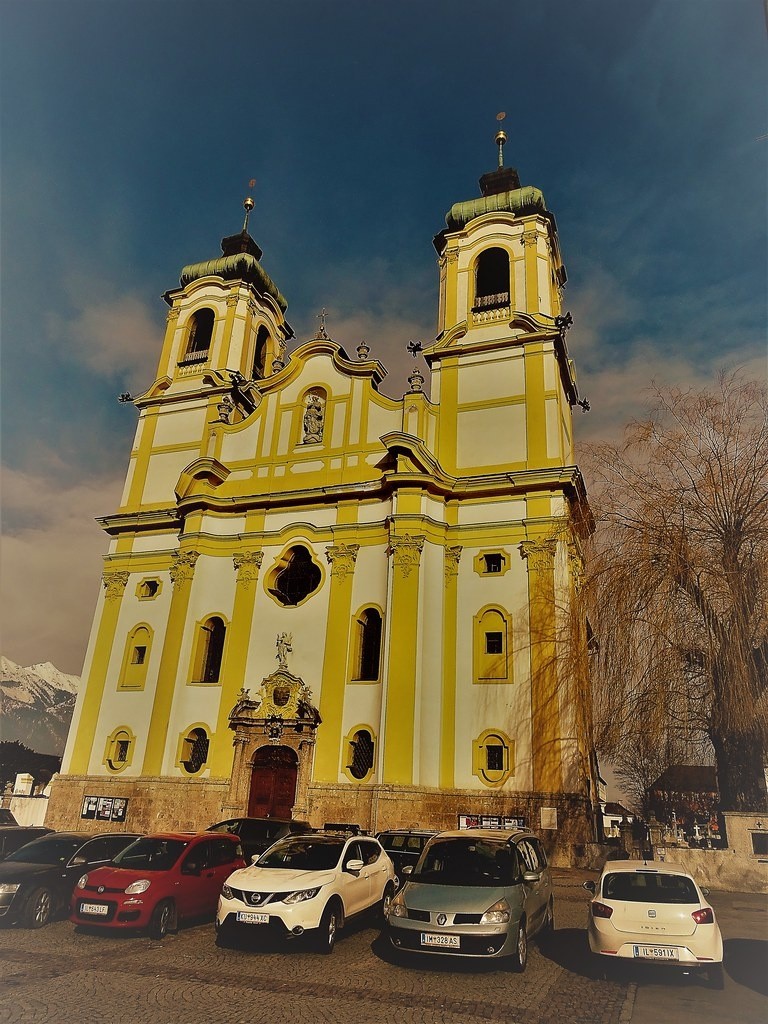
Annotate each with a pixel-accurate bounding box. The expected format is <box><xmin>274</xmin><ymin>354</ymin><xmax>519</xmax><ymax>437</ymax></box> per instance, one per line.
<box><xmin>581</xmin><ymin>859</ymin><xmax>725</xmax><ymax>990</ymax></box>
<box><xmin>70</xmin><ymin>830</ymin><xmax>247</xmax><ymax>940</ymax></box>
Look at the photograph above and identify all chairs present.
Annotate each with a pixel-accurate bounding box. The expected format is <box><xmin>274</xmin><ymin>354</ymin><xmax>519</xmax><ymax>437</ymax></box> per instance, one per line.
<box><xmin>496</xmin><ymin>849</ymin><xmax>509</xmax><ymax>863</ymax></box>
<box><xmin>661</xmin><ymin>876</ymin><xmax>683</xmax><ymax>899</ymax></box>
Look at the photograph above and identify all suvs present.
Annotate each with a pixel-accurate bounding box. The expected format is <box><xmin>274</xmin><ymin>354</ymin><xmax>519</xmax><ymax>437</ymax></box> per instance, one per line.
<box><xmin>205</xmin><ymin>817</ymin><xmax>313</xmax><ymax>867</ymax></box>
<box><xmin>213</xmin><ymin>822</ymin><xmax>396</xmax><ymax>955</ymax></box>
<box><xmin>374</xmin><ymin>829</ymin><xmax>446</xmax><ymax>896</ymax></box>
<box><xmin>385</xmin><ymin>829</ymin><xmax>554</xmax><ymax>973</ymax></box>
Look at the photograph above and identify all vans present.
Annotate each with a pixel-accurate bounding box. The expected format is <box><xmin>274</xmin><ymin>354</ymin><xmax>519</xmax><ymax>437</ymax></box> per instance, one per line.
<box><xmin>0</xmin><ymin>831</ymin><xmax>167</xmax><ymax>929</ymax></box>
